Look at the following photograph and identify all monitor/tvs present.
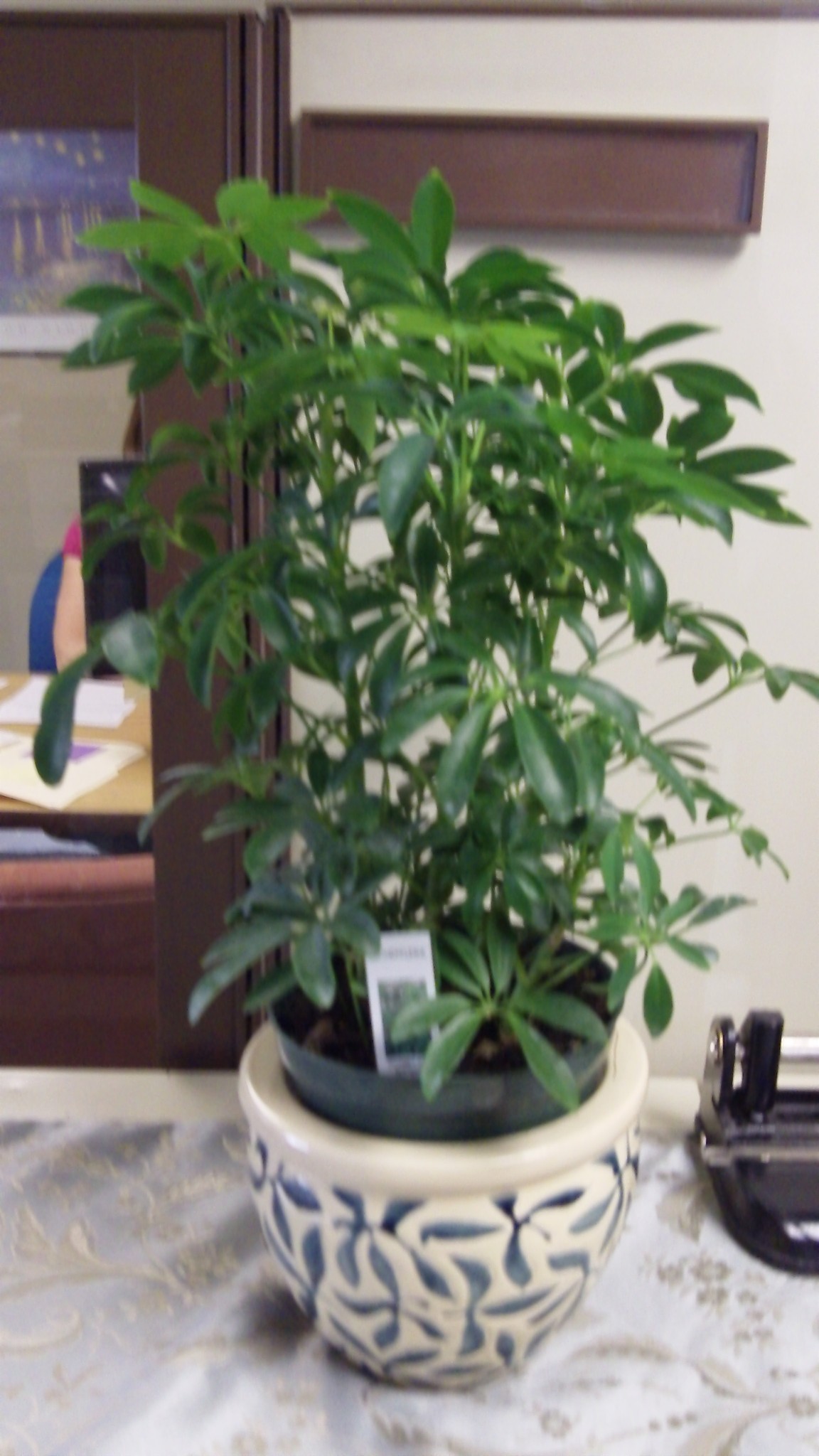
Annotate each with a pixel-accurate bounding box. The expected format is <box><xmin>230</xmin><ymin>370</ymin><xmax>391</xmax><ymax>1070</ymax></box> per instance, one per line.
<box><xmin>76</xmin><ymin>457</ymin><xmax>148</xmax><ymax>679</ymax></box>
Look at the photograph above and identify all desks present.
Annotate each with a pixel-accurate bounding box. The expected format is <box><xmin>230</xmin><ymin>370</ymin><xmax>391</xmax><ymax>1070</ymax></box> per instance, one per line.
<box><xmin>0</xmin><ymin>671</ymin><xmax>153</xmax><ymax>851</ymax></box>
<box><xmin>0</xmin><ymin>1067</ymin><xmax>819</xmax><ymax>1456</ymax></box>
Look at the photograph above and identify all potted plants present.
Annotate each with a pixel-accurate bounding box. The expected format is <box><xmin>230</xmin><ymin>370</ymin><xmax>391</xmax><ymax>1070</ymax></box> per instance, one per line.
<box><xmin>29</xmin><ymin>160</ymin><xmax>819</xmax><ymax>1398</ymax></box>
